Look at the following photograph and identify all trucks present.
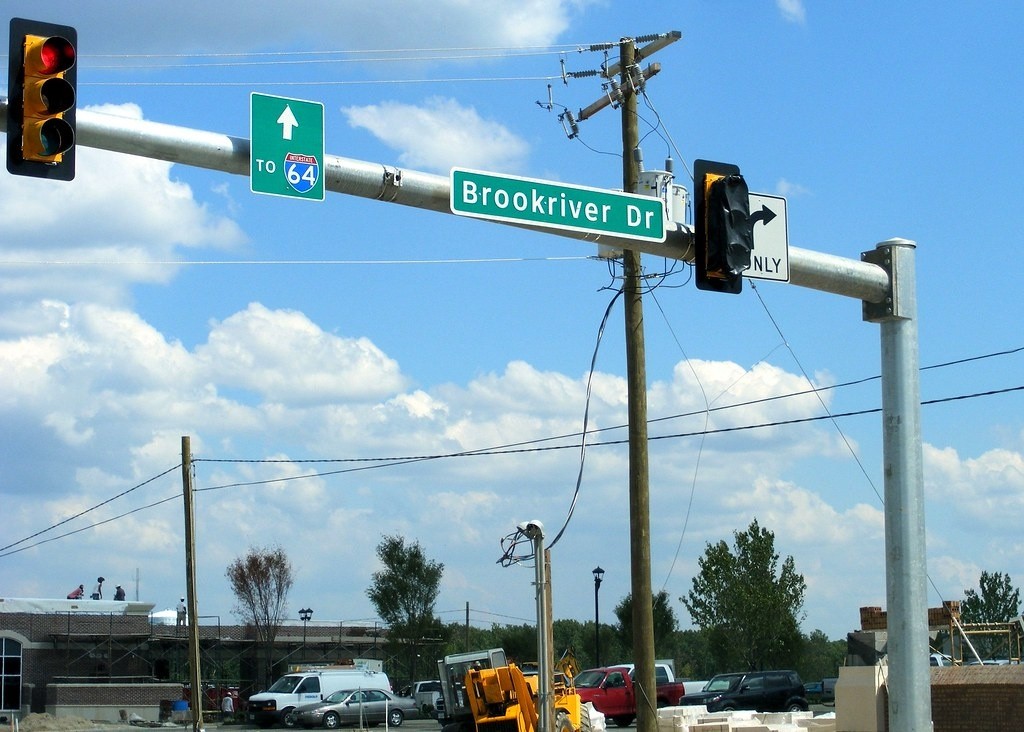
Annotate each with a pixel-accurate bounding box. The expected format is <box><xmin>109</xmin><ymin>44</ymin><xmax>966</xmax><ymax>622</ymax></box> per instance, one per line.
<box><xmin>581</xmin><ymin>664</ymin><xmax>729</xmax><ymax>705</ymax></box>
<box><xmin>248</xmin><ymin>669</ymin><xmax>393</xmax><ymax>727</ymax></box>
<box><xmin>411</xmin><ymin>681</ymin><xmax>464</xmax><ymax>713</ymax></box>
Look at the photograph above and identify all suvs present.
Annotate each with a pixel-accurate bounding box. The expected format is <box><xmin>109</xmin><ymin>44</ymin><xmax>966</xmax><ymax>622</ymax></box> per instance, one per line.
<box><xmin>680</xmin><ymin>671</ymin><xmax>809</xmax><ymax>712</ymax></box>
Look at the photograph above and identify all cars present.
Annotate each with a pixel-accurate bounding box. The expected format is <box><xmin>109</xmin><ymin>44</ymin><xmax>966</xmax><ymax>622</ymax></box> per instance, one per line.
<box><xmin>292</xmin><ymin>687</ymin><xmax>419</xmax><ymax>729</ymax></box>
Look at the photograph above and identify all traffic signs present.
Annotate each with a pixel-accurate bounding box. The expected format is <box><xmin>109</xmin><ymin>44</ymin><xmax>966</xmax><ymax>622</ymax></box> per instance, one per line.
<box><xmin>250</xmin><ymin>90</ymin><xmax>326</xmax><ymax>203</ymax></box>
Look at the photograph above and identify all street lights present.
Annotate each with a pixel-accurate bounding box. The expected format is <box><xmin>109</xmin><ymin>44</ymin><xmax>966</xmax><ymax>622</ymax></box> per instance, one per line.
<box><xmin>591</xmin><ymin>567</ymin><xmax>605</xmax><ymax>668</ymax></box>
<box><xmin>298</xmin><ymin>608</ymin><xmax>314</xmax><ymax>661</ymax></box>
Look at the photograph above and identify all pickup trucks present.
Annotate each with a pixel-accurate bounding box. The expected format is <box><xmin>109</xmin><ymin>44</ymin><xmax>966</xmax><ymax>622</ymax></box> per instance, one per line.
<box><xmin>567</xmin><ymin>667</ymin><xmax>685</xmax><ymax>727</ymax></box>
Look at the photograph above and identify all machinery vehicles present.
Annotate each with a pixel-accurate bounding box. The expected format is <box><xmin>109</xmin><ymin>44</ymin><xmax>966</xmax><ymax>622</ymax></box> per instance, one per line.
<box><xmin>436</xmin><ymin>645</ymin><xmax>581</xmax><ymax>732</ymax></box>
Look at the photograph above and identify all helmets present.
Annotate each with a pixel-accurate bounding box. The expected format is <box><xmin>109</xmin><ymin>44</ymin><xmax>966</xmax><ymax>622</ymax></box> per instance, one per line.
<box><xmin>180</xmin><ymin>597</ymin><xmax>185</xmax><ymax>600</ymax></box>
<box><xmin>115</xmin><ymin>584</ymin><xmax>121</xmax><ymax>587</ymax></box>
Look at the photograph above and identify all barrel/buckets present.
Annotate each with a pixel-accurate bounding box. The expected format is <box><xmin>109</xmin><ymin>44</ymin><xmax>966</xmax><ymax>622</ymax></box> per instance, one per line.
<box><xmin>173</xmin><ymin>700</ymin><xmax>188</xmax><ymax>711</ymax></box>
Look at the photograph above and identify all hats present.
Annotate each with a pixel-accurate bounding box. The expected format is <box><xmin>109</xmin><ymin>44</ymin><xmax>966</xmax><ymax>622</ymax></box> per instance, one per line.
<box><xmin>227</xmin><ymin>692</ymin><xmax>232</xmax><ymax>696</ymax></box>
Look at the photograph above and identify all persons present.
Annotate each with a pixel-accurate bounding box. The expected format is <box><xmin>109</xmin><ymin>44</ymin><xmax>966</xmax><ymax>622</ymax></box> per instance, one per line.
<box><xmin>90</xmin><ymin>577</ymin><xmax>105</xmax><ymax>600</ymax></box>
<box><xmin>221</xmin><ymin>692</ymin><xmax>234</xmax><ymax>718</ymax></box>
<box><xmin>67</xmin><ymin>584</ymin><xmax>83</xmax><ymax>599</ymax></box>
<box><xmin>176</xmin><ymin>597</ymin><xmax>186</xmax><ymax>625</ymax></box>
<box><xmin>114</xmin><ymin>584</ymin><xmax>125</xmax><ymax>600</ymax></box>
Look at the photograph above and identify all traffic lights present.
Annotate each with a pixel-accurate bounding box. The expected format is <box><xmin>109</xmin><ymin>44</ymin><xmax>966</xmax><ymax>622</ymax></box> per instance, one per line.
<box><xmin>22</xmin><ymin>35</ymin><xmax>75</xmax><ymax>162</ymax></box>
<box><xmin>704</xmin><ymin>173</ymin><xmax>753</xmax><ymax>280</ymax></box>
